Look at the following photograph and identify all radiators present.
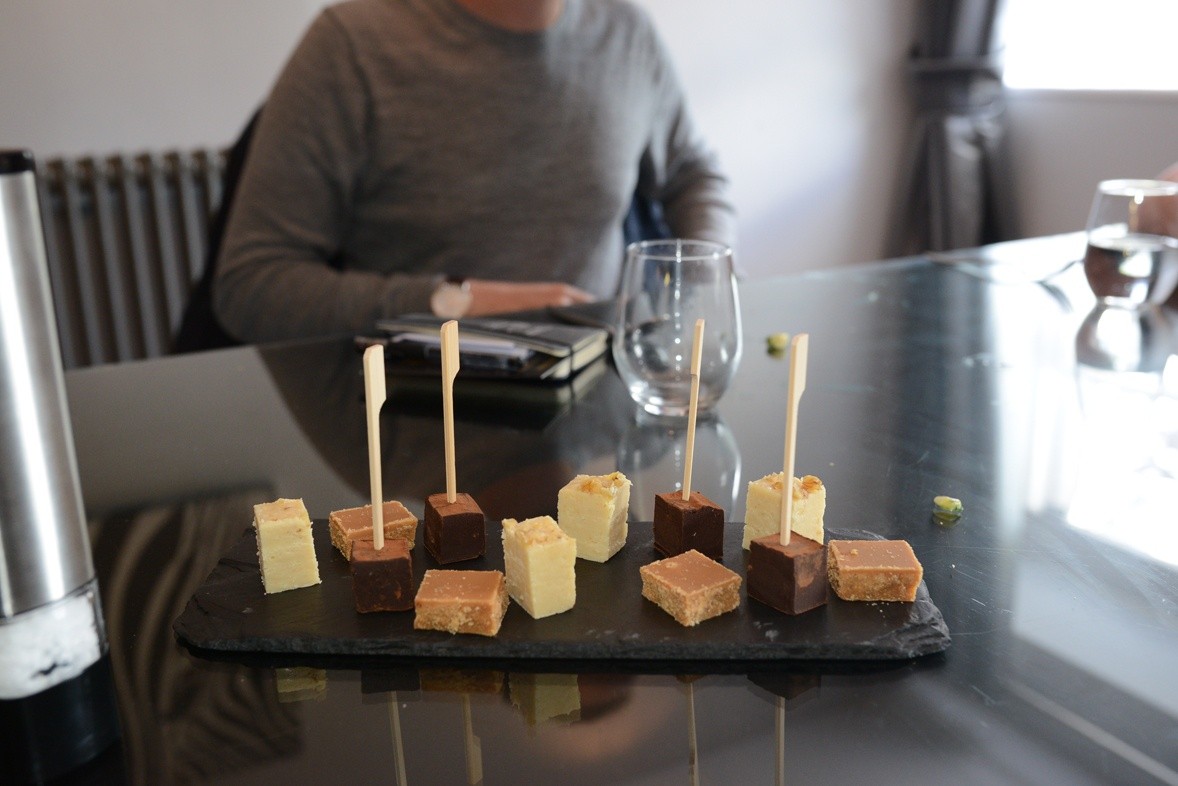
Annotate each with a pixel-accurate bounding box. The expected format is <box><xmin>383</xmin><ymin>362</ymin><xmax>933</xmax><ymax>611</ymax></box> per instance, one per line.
<box><xmin>36</xmin><ymin>142</ymin><xmax>229</xmax><ymax>373</ymax></box>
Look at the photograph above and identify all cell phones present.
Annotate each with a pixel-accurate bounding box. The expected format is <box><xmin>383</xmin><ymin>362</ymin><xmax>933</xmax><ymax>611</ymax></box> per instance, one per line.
<box><xmin>389</xmin><ymin>330</ymin><xmax>534</xmax><ymax>370</ymax></box>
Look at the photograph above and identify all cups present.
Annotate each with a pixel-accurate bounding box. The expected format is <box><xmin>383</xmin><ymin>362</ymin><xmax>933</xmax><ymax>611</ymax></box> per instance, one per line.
<box><xmin>1085</xmin><ymin>178</ymin><xmax>1178</xmax><ymax>310</ymax></box>
<box><xmin>615</xmin><ymin>238</ymin><xmax>744</xmax><ymax>416</ymax></box>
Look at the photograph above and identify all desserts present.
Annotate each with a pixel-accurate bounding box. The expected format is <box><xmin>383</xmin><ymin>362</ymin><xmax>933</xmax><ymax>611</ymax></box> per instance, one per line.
<box><xmin>236</xmin><ymin>467</ymin><xmax>926</xmax><ymax>637</ymax></box>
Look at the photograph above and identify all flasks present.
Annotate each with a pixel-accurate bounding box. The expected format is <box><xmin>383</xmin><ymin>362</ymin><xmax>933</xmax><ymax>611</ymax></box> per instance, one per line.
<box><xmin>2</xmin><ymin>146</ymin><xmax>114</xmax><ymax>702</ymax></box>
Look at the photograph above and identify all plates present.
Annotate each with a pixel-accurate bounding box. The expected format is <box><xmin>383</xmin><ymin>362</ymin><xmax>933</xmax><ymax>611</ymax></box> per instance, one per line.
<box><xmin>172</xmin><ymin>513</ymin><xmax>956</xmax><ymax>665</ymax></box>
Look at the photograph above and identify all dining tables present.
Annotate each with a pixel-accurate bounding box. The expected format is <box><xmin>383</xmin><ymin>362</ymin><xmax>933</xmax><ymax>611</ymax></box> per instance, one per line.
<box><xmin>59</xmin><ymin>223</ymin><xmax>1178</xmax><ymax>786</ymax></box>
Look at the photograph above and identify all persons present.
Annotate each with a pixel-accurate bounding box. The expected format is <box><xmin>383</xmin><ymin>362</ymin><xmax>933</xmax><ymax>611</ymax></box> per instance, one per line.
<box><xmin>204</xmin><ymin>1</ymin><xmax>739</xmax><ymax>341</ymax></box>
